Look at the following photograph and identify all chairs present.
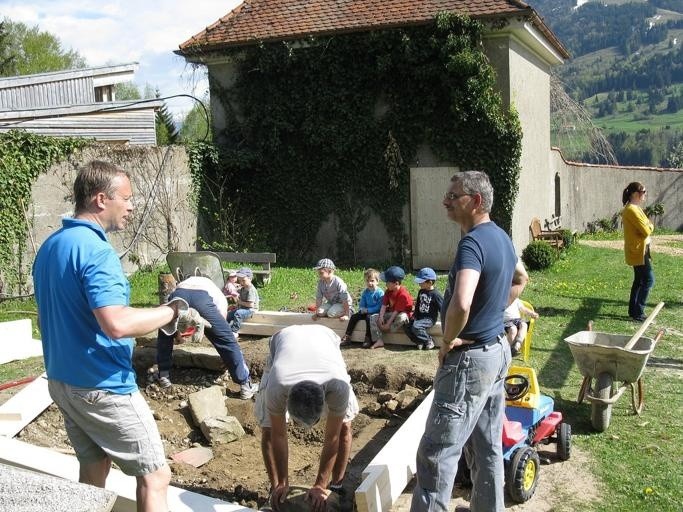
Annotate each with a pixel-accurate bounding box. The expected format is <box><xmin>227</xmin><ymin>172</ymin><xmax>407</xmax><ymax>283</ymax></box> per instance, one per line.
<box><xmin>517</xmin><ymin>300</ymin><xmax>535</xmax><ymax>362</ymax></box>
<box><xmin>502</xmin><ymin>412</ymin><xmax>526</xmax><ymax>448</ymax></box>
<box><xmin>529</xmin><ymin>218</ymin><xmax>564</xmax><ymax>260</ymax></box>
<box><xmin>544</xmin><ymin>214</ymin><xmax>578</xmax><ymax>247</ymax></box>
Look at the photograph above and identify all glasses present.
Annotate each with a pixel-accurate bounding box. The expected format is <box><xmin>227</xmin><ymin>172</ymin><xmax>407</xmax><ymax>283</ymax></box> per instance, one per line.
<box><xmin>443</xmin><ymin>192</ymin><xmax>473</xmax><ymax>200</ymax></box>
<box><xmin>637</xmin><ymin>190</ymin><xmax>646</xmax><ymax>194</ymax></box>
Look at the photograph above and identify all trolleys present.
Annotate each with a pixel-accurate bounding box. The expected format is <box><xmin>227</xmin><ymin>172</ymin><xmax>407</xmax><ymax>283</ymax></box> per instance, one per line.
<box><xmin>563</xmin><ymin>320</ymin><xmax>666</xmax><ymax>432</ymax></box>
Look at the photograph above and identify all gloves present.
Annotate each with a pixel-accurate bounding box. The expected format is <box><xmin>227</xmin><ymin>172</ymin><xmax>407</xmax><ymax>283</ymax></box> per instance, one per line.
<box><xmin>159</xmin><ymin>297</ymin><xmax>212</xmax><ymax>344</ymax></box>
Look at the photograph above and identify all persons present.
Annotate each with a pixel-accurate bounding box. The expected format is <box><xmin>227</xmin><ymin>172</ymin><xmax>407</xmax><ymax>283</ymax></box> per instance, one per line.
<box><xmin>309</xmin><ymin>259</ymin><xmax>349</xmax><ymax>315</ymax></box>
<box><xmin>502</xmin><ymin>290</ymin><xmax>539</xmax><ymax>355</ymax></box>
<box><xmin>257</xmin><ymin>325</ymin><xmax>357</xmax><ymax>511</ymax></box>
<box><xmin>224</xmin><ymin>272</ymin><xmax>240</xmax><ymax>297</ymax></box>
<box><xmin>620</xmin><ymin>182</ymin><xmax>655</xmax><ymax>322</ymax></box>
<box><xmin>340</xmin><ymin>267</ymin><xmax>384</xmax><ymax>348</ymax></box>
<box><xmin>369</xmin><ymin>265</ymin><xmax>415</xmax><ymax>350</ymax></box>
<box><xmin>405</xmin><ymin>267</ymin><xmax>446</xmax><ymax>351</ymax></box>
<box><xmin>32</xmin><ymin>159</ymin><xmax>211</xmax><ymax>511</ymax></box>
<box><xmin>149</xmin><ymin>277</ymin><xmax>260</xmax><ymax>403</ymax></box>
<box><xmin>226</xmin><ymin>269</ymin><xmax>260</xmax><ymax>338</ymax></box>
<box><xmin>409</xmin><ymin>170</ymin><xmax>528</xmax><ymax>512</ymax></box>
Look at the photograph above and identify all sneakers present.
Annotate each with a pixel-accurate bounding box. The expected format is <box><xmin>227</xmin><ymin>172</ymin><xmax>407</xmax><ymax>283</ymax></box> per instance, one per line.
<box><xmin>240</xmin><ymin>383</ymin><xmax>258</xmax><ymax>399</ymax></box>
<box><xmin>634</xmin><ymin>314</ymin><xmax>647</xmax><ymax>321</ymax></box>
<box><xmin>157</xmin><ymin>374</ymin><xmax>171</xmax><ymax>388</ymax></box>
<box><xmin>340</xmin><ymin>335</ymin><xmax>351</xmax><ymax>346</ymax></box>
<box><xmin>511</xmin><ymin>344</ymin><xmax>521</xmax><ymax>357</ymax></box>
<box><xmin>362</xmin><ymin>337</ymin><xmax>373</xmax><ymax>347</ymax></box>
<box><xmin>417</xmin><ymin>343</ymin><xmax>424</xmax><ymax>350</ymax></box>
<box><xmin>425</xmin><ymin>341</ymin><xmax>435</xmax><ymax>349</ymax></box>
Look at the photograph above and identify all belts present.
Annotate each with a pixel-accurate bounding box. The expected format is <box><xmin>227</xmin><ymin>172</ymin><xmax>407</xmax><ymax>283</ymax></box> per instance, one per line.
<box><xmin>460</xmin><ymin>332</ymin><xmax>504</xmax><ymax>348</ymax></box>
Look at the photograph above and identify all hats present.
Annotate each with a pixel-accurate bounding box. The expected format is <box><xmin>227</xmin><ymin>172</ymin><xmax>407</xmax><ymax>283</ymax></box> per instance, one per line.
<box><xmin>379</xmin><ymin>265</ymin><xmax>405</xmax><ymax>281</ymax></box>
<box><xmin>235</xmin><ymin>268</ymin><xmax>253</xmax><ymax>278</ymax></box>
<box><xmin>413</xmin><ymin>267</ymin><xmax>436</xmax><ymax>283</ymax></box>
<box><xmin>313</xmin><ymin>259</ymin><xmax>336</xmax><ymax>271</ymax></box>
<box><xmin>229</xmin><ymin>269</ymin><xmax>236</xmax><ymax>277</ymax></box>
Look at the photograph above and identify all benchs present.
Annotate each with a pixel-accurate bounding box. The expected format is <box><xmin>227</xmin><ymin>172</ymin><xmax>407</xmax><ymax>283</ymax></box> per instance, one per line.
<box><xmin>217</xmin><ymin>252</ymin><xmax>276</xmax><ymax>286</ymax></box>
